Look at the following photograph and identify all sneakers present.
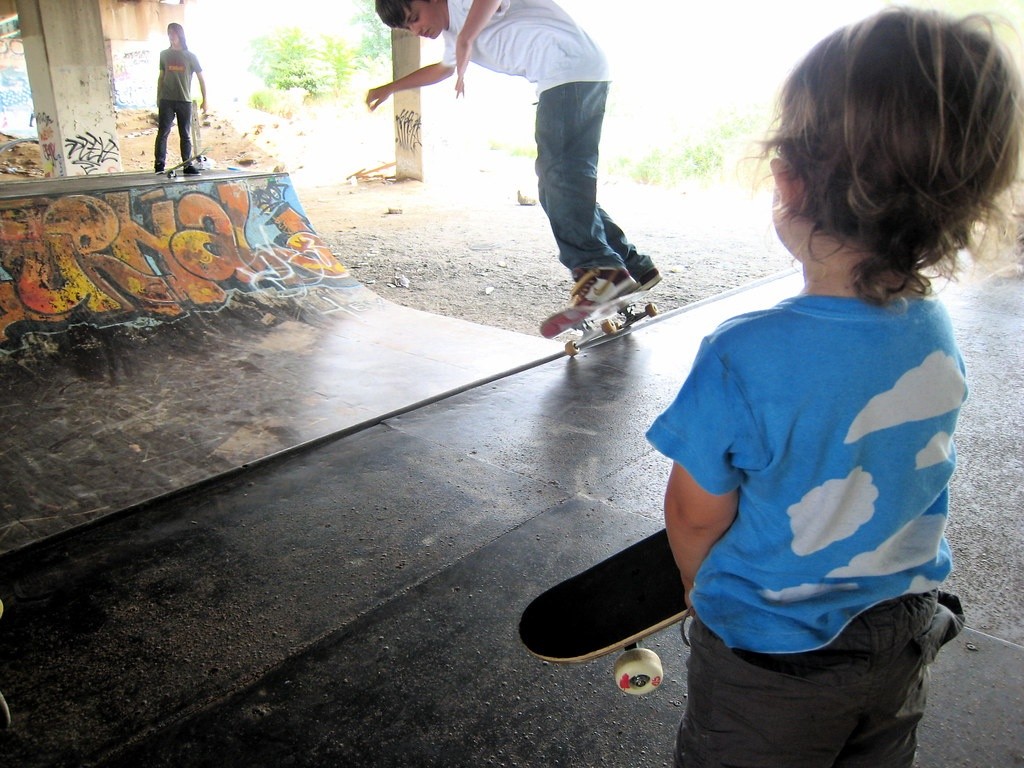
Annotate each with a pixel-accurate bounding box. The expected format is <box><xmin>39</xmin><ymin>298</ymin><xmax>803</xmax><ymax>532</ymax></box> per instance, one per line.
<box><xmin>569</xmin><ymin>263</ymin><xmax>662</xmax><ymax>308</ymax></box>
<box><xmin>154</xmin><ymin>163</ymin><xmax>165</xmax><ymax>175</ymax></box>
<box><xmin>182</xmin><ymin>165</ymin><xmax>201</xmax><ymax>176</ymax></box>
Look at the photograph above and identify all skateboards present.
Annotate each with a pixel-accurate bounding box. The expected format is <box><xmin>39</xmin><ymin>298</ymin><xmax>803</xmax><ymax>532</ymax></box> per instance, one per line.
<box><xmin>538</xmin><ymin>287</ymin><xmax>659</xmax><ymax>357</ymax></box>
<box><xmin>516</xmin><ymin>528</ymin><xmax>689</xmax><ymax>697</ymax></box>
<box><xmin>158</xmin><ymin>145</ymin><xmax>211</xmax><ymax>178</ymax></box>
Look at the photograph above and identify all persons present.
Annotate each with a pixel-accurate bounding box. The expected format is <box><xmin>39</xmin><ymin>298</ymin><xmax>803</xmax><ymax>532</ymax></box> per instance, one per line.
<box><xmin>153</xmin><ymin>22</ymin><xmax>208</xmax><ymax>176</ymax></box>
<box><xmin>639</xmin><ymin>5</ymin><xmax>1024</xmax><ymax>766</ymax></box>
<box><xmin>363</xmin><ymin>1</ymin><xmax>666</xmax><ymax>315</ymax></box>
